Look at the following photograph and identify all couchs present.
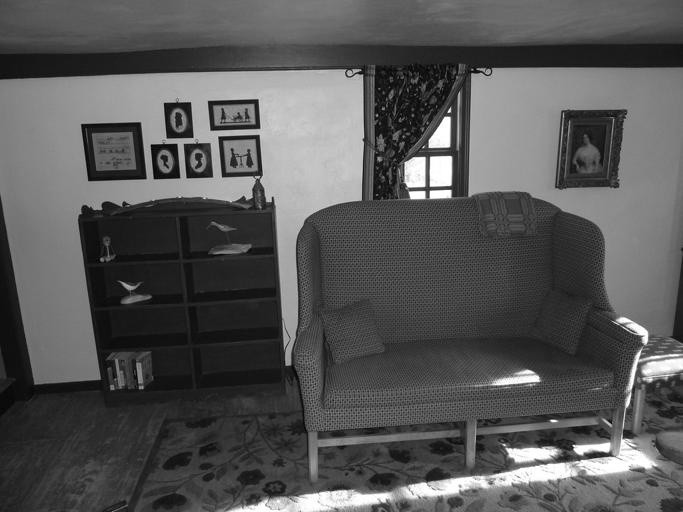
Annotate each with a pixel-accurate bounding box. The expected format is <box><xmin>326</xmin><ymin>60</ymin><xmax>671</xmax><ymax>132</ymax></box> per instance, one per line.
<box><xmin>294</xmin><ymin>192</ymin><xmax>649</xmax><ymax>485</ymax></box>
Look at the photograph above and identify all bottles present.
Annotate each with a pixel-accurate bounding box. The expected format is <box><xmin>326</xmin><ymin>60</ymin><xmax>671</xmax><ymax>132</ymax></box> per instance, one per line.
<box><xmin>252</xmin><ymin>171</ymin><xmax>266</xmax><ymax>210</ymax></box>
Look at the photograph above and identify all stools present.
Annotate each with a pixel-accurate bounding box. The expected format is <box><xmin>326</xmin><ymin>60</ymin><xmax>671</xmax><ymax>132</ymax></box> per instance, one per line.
<box><xmin>632</xmin><ymin>333</ymin><xmax>683</xmax><ymax>435</ymax></box>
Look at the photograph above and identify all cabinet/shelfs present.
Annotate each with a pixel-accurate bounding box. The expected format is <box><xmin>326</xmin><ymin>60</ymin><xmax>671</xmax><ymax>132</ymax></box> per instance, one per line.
<box><xmin>79</xmin><ymin>196</ymin><xmax>287</xmax><ymax>408</ymax></box>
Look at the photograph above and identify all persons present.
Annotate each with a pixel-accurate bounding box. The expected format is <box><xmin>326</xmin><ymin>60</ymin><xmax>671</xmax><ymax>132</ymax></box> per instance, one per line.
<box><xmin>571</xmin><ymin>132</ymin><xmax>602</xmax><ymax>174</ymax></box>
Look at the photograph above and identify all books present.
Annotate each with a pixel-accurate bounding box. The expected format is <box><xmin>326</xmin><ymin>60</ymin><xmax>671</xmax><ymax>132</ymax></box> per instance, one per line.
<box><xmin>104</xmin><ymin>350</ymin><xmax>155</xmax><ymax>393</ymax></box>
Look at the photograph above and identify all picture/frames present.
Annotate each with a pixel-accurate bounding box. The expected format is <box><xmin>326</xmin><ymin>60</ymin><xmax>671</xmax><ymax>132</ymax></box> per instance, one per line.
<box><xmin>218</xmin><ymin>135</ymin><xmax>263</xmax><ymax>177</ymax></box>
<box><xmin>556</xmin><ymin>109</ymin><xmax>627</xmax><ymax>190</ymax></box>
<box><xmin>208</xmin><ymin>99</ymin><xmax>260</xmax><ymax>131</ymax></box>
<box><xmin>81</xmin><ymin>122</ymin><xmax>147</xmax><ymax>181</ymax></box>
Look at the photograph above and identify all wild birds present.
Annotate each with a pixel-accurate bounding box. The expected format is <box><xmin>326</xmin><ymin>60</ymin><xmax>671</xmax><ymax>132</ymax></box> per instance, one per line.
<box><xmin>116</xmin><ymin>280</ymin><xmax>144</xmax><ymax>296</ymax></box>
<box><xmin>206</xmin><ymin>221</ymin><xmax>239</xmax><ymax>243</ymax></box>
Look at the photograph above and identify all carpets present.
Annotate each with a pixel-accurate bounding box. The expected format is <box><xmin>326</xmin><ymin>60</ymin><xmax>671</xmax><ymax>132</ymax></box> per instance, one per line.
<box><xmin>128</xmin><ymin>378</ymin><xmax>683</xmax><ymax>512</ymax></box>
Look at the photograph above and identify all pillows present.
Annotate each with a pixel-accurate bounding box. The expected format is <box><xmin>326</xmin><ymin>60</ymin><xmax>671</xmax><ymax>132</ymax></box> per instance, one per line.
<box><xmin>528</xmin><ymin>289</ymin><xmax>592</xmax><ymax>357</ymax></box>
<box><xmin>319</xmin><ymin>299</ymin><xmax>385</xmax><ymax>365</ymax></box>
